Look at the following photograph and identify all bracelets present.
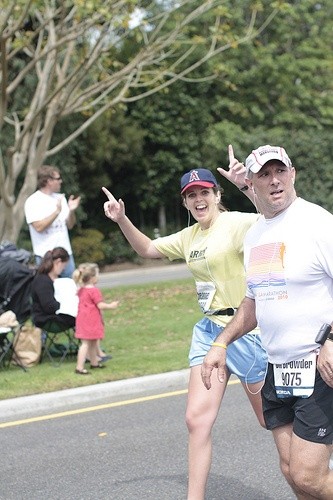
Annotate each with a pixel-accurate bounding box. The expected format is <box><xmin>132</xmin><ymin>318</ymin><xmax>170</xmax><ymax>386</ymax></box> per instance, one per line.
<box><xmin>240</xmin><ymin>185</ymin><xmax>249</xmax><ymax>191</ymax></box>
<box><xmin>211</xmin><ymin>343</ymin><xmax>226</xmax><ymax>349</ymax></box>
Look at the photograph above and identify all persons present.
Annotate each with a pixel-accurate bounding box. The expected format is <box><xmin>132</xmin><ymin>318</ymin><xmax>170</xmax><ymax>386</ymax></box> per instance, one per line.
<box><xmin>72</xmin><ymin>264</ymin><xmax>119</xmax><ymax>374</ymax></box>
<box><xmin>201</xmin><ymin>144</ymin><xmax>333</xmax><ymax>500</ymax></box>
<box><xmin>102</xmin><ymin>144</ymin><xmax>268</xmax><ymax>500</ymax></box>
<box><xmin>24</xmin><ymin>165</ymin><xmax>81</xmax><ymax>278</ymax></box>
<box><xmin>31</xmin><ymin>247</ymin><xmax>111</xmax><ymax>362</ymax></box>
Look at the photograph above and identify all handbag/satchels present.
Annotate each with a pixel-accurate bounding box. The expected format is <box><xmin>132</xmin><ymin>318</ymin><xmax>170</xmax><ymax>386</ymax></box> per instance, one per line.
<box><xmin>11</xmin><ymin>327</ymin><xmax>42</xmax><ymax>367</ymax></box>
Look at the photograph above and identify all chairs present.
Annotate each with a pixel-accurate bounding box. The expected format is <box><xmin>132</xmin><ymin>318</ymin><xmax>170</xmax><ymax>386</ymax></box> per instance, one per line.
<box><xmin>0</xmin><ymin>293</ymin><xmax>80</xmax><ymax>373</ymax></box>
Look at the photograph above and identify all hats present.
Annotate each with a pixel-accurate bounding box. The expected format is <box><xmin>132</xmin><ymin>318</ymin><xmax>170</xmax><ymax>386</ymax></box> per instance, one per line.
<box><xmin>245</xmin><ymin>144</ymin><xmax>292</xmax><ymax>178</ymax></box>
<box><xmin>179</xmin><ymin>169</ymin><xmax>219</xmax><ymax>194</ymax></box>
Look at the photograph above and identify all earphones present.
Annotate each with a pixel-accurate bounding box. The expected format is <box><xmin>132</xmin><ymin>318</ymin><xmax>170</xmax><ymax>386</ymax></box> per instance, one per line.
<box><xmin>252</xmin><ymin>184</ymin><xmax>255</xmax><ymax>193</ymax></box>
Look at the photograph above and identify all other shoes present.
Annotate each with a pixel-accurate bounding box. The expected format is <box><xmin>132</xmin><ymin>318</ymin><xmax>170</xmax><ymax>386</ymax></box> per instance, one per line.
<box><xmin>101</xmin><ymin>355</ymin><xmax>112</xmax><ymax>359</ymax></box>
<box><xmin>76</xmin><ymin>369</ymin><xmax>88</xmax><ymax>374</ymax></box>
<box><xmin>91</xmin><ymin>364</ymin><xmax>106</xmax><ymax>368</ymax></box>
